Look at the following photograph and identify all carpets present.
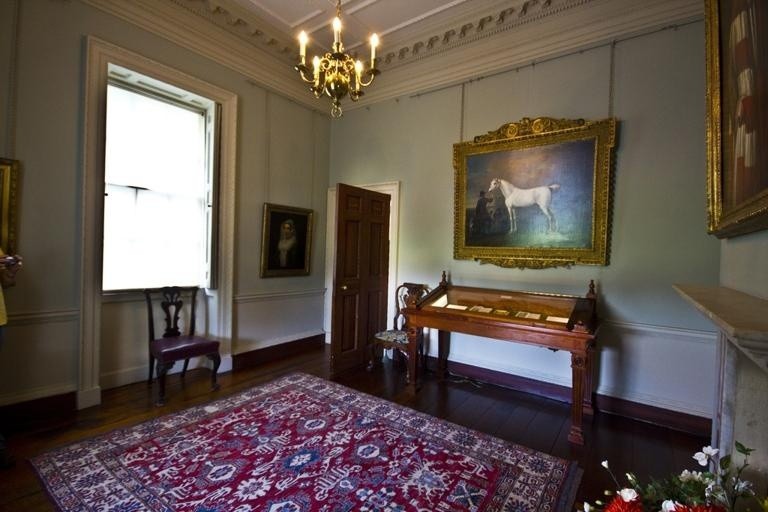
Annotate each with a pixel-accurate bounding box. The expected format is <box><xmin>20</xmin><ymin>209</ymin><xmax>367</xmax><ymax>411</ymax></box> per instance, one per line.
<box><xmin>24</xmin><ymin>369</ymin><xmax>572</xmax><ymax>512</ymax></box>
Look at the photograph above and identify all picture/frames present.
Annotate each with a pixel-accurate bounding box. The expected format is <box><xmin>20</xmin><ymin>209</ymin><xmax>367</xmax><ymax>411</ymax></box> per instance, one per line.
<box><xmin>0</xmin><ymin>155</ymin><xmax>20</xmax><ymax>257</ymax></box>
<box><xmin>703</xmin><ymin>0</ymin><xmax>768</xmax><ymax>240</ymax></box>
<box><xmin>259</xmin><ymin>202</ymin><xmax>314</xmax><ymax>279</ymax></box>
<box><xmin>452</xmin><ymin>116</ymin><xmax>618</xmax><ymax>269</ymax></box>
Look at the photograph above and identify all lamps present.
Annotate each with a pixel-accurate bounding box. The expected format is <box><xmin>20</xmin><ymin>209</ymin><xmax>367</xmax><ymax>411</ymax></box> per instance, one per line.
<box><xmin>293</xmin><ymin>0</ymin><xmax>383</xmax><ymax>120</ymax></box>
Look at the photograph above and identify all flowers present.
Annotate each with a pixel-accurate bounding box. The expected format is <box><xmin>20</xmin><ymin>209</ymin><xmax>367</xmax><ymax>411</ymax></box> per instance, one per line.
<box><xmin>583</xmin><ymin>441</ymin><xmax>768</xmax><ymax>512</ymax></box>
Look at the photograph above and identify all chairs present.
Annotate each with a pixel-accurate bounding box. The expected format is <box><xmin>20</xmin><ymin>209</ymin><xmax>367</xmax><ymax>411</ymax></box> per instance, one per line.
<box><xmin>370</xmin><ymin>282</ymin><xmax>430</xmax><ymax>385</ymax></box>
<box><xmin>143</xmin><ymin>286</ymin><xmax>222</xmax><ymax>409</ymax></box>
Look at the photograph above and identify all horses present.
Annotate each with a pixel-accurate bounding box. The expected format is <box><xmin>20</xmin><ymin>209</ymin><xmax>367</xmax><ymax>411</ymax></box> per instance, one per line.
<box><xmin>488</xmin><ymin>176</ymin><xmax>560</xmax><ymax>235</ymax></box>
